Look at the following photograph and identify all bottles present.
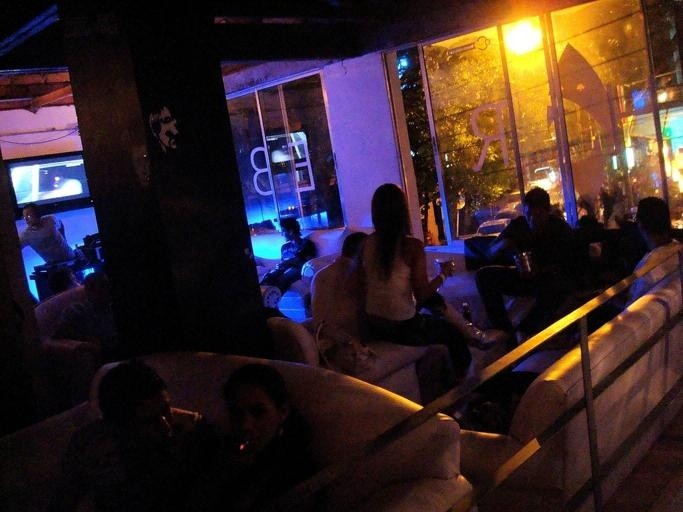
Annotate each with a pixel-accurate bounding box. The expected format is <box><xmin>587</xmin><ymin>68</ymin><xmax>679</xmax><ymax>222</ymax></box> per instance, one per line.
<box><xmin>462</xmin><ymin>303</ymin><xmax>472</xmax><ymax>326</ymax></box>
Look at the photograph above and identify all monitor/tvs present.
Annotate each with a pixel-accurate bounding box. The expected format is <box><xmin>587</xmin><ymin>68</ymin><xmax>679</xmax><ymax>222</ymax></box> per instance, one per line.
<box><xmin>5</xmin><ymin>152</ymin><xmax>91</xmax><ymax>211</ymax></box>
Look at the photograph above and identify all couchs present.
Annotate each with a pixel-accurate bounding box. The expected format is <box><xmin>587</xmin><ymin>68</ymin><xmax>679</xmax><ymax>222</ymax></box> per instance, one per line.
<box><xmin>1</xmin><ymin>225</ymin><xmax>683</xmax><ymax>512</ymax></box>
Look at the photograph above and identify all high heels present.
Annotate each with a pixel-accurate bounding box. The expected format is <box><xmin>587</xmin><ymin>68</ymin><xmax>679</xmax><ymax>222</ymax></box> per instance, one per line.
<box><xmin>459</xmin><ymin>323</ymin><xmax>504</xmax><ymax>355</ymax></box>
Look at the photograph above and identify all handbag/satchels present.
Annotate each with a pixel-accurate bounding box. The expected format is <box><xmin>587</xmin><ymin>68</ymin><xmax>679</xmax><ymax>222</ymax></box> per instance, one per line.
<box><xmin>314</xmin><ymin>320</ymin><xmax>375</xmax><ymax>373</ymax></box>
